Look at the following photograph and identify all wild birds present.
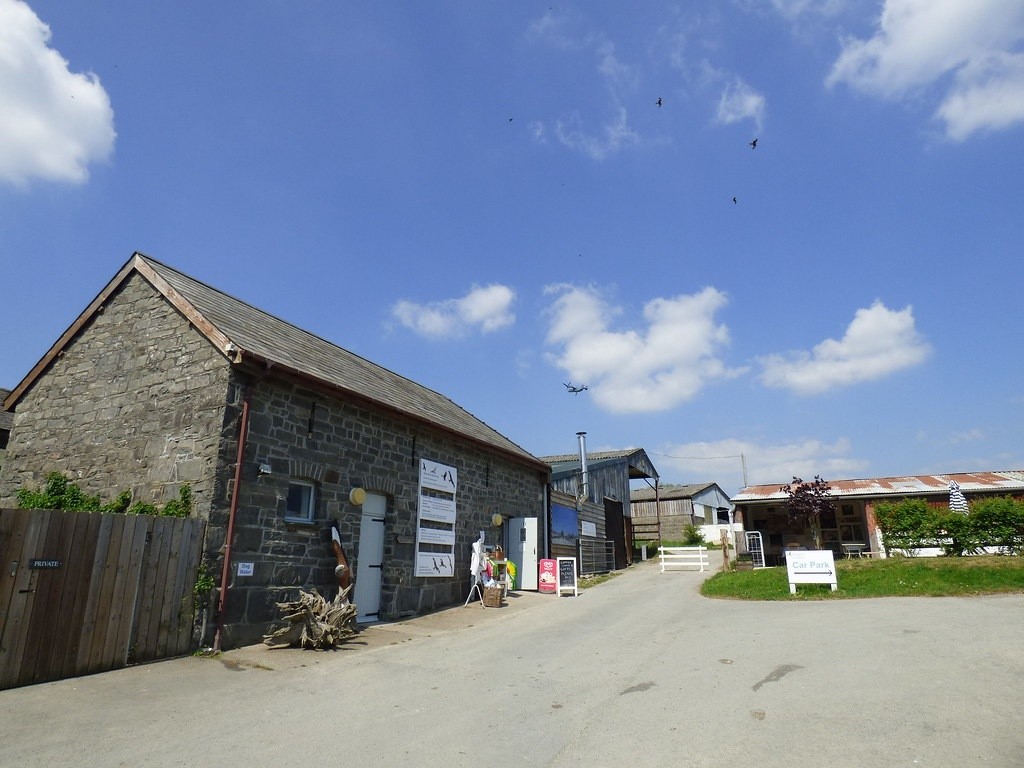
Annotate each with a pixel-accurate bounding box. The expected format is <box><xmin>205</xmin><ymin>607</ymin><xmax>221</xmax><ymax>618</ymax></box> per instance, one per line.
<box><xmin>733</xmin><ymin>197</ymin><xmax>737</xmax><ymax>205</ymax></box>
<box><xmin>655</xmin><ymin>98</ymin><xmax>662</xmax><ymax>108</ymax></box>
<box><xmin>563</xmin><ymin>381</ymin><xmax>588</xmax><ymax>396</ymax></box>
<box><xmin>509</xmin><ymin>118</ymin><xmax>514</xmax><ymax>122</ymax></box>
<box><xmin>748</xmin><ymin>138</ymin><xmax>758</xmax><ymax>150</ymax></box>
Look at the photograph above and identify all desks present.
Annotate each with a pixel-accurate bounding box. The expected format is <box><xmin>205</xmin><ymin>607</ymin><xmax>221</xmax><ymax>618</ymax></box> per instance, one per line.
<box><xmin>841</xmin><ymin>543</ymin><xmax>866</xmax><ymax>560</ymax></box>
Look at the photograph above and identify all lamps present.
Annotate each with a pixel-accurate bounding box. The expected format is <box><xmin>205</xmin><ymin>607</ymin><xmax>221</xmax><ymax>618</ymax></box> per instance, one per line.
<box><xmin>257</xmin><ymin>462</ymin><xmax>272</xmax><ymax>475</ymax></box>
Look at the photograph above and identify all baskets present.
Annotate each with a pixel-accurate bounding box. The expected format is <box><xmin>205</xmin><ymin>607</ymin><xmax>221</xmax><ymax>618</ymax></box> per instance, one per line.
<box><xmin>483</xmin><ymin>583</ymin><xmax>504</xmax><ymax>607</ymax></box>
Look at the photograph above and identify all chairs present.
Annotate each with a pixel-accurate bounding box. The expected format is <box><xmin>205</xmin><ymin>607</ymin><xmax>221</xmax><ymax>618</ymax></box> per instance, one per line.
<box><xmin>786</xmin><ymin>542</ymin><xmax>799</xmax><ymax>551</ymax></box>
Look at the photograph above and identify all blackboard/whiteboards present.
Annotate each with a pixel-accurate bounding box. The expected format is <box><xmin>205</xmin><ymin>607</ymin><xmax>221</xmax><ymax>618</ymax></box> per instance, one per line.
<box><xmin>556</xmin><ymin>557</ymin><xmax>577</xmax><ymax>590</ymax></box>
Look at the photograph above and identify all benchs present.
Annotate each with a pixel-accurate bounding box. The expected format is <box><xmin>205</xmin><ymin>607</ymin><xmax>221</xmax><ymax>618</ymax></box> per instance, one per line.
<box><xmin>861</xmin><ymin>550</ymin><xmax>897</xmax><ymax>559</ymax></box>
